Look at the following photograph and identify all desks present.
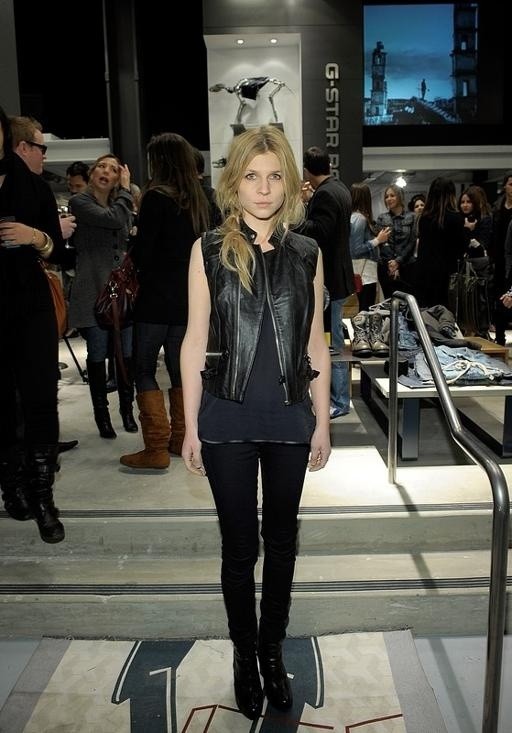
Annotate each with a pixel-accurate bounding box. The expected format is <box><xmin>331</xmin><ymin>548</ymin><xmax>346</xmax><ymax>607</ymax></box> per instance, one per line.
<box><xmin>315</xmin><ymin>337</ymin><xmax>506</xmax><ymax>398</ymax></box>
<box><xmin>361</xmin><ymin>361</ymin><xmax>512</xmax><ymax>461</ymax></box>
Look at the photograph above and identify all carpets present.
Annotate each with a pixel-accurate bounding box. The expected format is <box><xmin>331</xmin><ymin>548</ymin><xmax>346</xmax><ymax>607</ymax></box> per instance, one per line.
<box><xmin>0</xmin><ymin>628</ymin><xmax>448</xmax><ymax>733</ymax></box>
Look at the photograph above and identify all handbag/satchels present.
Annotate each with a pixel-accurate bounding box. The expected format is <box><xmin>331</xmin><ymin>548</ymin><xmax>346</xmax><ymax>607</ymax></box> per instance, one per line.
<box><xmin>450</xmin><ymin>245</ymin><xmax>497</xmax><ymax>334</ymax></box>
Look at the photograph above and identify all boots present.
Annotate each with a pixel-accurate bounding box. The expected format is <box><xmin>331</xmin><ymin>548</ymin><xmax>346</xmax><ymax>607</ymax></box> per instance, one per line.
<box><xmin>29</xmin><ymin>451</ymin><xmax>65</xmax><ymax>544</ymax></box>
<box><xmin>114</xmin><ymin>356</ymin><xmax>140</xmax><ymax>433</ymax></box>
<box><xmin>119</xmin><ymin>391</ymin><xmax>170</xmax><ymax>470</ymax></box>
<box><xmin>349</xmin><ymin>313</ymin><xmax>372</xmax><ymax>357</ymax></box>
<box><xmin>368</xmin><ymin>313</ymin><xmax>391</xmax><ymax>356</ymax></box>
<box><xmin>1</xmin><ymin>439</ymin><xmax>33</xmax><ymax>520</ymax></box>
<box><xmin>168</xmin><ymin>387</ymin><xmax>186</xmax><ymax>455</ymax></box>
<box><xmin>85</xmin><ymin>360</ymin><xmax>119</xmax><ymax>439</ymax></box>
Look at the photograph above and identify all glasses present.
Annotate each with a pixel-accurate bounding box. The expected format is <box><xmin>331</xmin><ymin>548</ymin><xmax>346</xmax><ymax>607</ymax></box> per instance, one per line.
<box><xmin>26</xmin><ymin>139</ymin><xmax>48</xmax><ymax>155</ymax></box>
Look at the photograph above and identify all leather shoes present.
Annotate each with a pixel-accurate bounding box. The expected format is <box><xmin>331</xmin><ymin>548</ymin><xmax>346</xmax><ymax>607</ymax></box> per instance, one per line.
<box><xmin>59</xmin><ymin>440</ymin><xmax>78</xmax><ymax>452</ymax></box>
<box><xmin>259</xmin><ymin>642</ymin><xmax>295</xmax><ymax>711</ymax></box>
<box><xmin>233</xmin><ymin>644</ymin><xmax>263</xmax><ymax>718</ymax></box>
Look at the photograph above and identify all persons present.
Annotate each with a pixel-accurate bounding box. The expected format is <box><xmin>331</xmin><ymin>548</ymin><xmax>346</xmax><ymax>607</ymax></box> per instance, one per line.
<box><xmin>180</xmin><ymin>125</ymin><xmax>331</xmax><ymax>721</ymax></box>
<box><xmin>288</xmin><ymin>144</ymin><xmax>512</xmax><ymax>418</ymax></box>
<box><xmin>421</xmin><ymin>78</ymin><xmax>426</xmax><ymax>101</ymax></box>
<box><xmin>1</xmin><ymin>115</ymin><xmax>221</xmax><ymax>541</ymax></box>
